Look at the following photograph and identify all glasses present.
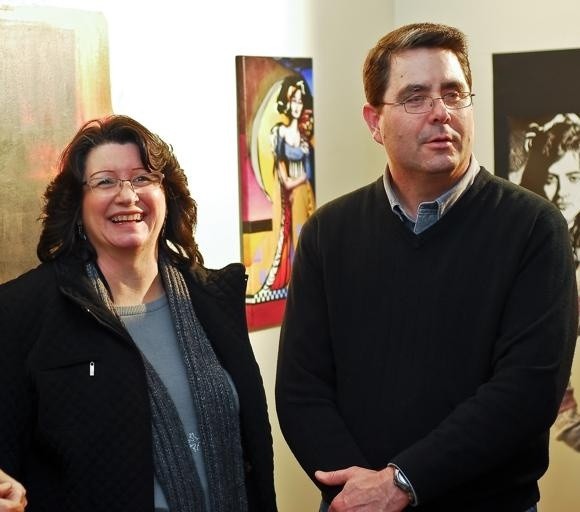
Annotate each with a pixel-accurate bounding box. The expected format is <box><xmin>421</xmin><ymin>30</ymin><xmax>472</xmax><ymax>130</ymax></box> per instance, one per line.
<box><xmin>385</xmin><ymin>90</ymin><xmax>475</xmax><ymax>115</ymax></box>
<box><xmin>82</xmin><ymin>169</ymin><xmax>163</xmax><ymax>199</ymax></box>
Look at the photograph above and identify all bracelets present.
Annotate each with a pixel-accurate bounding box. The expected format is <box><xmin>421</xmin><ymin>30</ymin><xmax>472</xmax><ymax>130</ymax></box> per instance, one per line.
<box><xmin>390</xmin><ymin>466</ymin><xmax>412</xmax><ymax>492</ymax></box>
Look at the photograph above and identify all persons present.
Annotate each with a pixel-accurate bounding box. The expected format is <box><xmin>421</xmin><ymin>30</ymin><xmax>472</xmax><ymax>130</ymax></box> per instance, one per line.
<box><xmin>1</xmin><ymin>115</ymin><xmax>277</xmax><ymax>512</ymax></box>
<box><xmin>499</xmin><ymin>109</ymin><xmax>579</xmax><ymax>265</ymax></box>
<box><xmin>272</xmin><ymin>22</ymin><xmax>580</xmax><ymax>511</ymax></box>
<box><xmin>1</xmin><ymin>469</ymin><xmax>27</xmax><ymax>512</ymax></box>
<box><xmin>262</xmin><ymin>65</ymin><xmax>315</xmax><ymax>299</ymax></box>
